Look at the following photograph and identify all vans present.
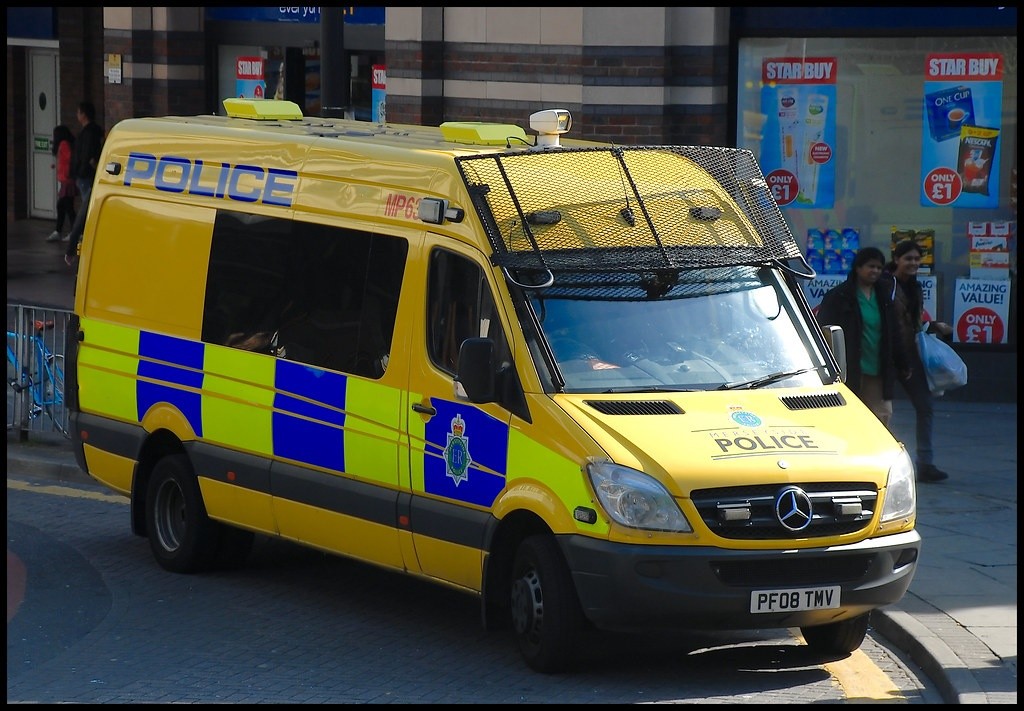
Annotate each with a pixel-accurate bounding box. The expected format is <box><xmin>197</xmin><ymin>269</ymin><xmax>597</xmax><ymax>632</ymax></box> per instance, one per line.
<box><xmin>61</xmin><ymin>100</ymin><xmax>922</xmax><ymax>679</ymax></box>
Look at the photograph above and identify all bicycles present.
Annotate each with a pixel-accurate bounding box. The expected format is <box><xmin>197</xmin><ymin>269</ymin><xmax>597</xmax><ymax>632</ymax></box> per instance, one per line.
<box><xmin>6</xmin><ymin>320</ymin><xmax>71</xmax><ymax>440</ymax></box>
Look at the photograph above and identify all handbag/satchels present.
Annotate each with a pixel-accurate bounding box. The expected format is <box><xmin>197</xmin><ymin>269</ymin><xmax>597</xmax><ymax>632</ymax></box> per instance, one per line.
<box><xmin>917</xmin><ymin>331</ymin><xmax>968</xmax><ymax>398</ymax></box>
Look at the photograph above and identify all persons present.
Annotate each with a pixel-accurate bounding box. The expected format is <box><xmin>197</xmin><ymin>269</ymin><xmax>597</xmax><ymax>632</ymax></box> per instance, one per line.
<box><xmin>73</xmin><ymin>98</ymin><xmax>103</xmax><ymax>250</ymax></box>
<box><xmin>64</xmin><ymin>146</ymin><xmax>102</xmax><ymax>265</ymax></box>
<box><xmin>45</xmin><ymin>124</ymin><xmax>77</xmax><ymax>242</ymax></box>
<box><xmin>815</xmin><ymin>246</ymin><xmax>894</xmax><ymax>429</ymax></box>
<box><xmin>877</xmin><ymin>240</ymin><xmax>951</xmax><ymax>481</ymax></box>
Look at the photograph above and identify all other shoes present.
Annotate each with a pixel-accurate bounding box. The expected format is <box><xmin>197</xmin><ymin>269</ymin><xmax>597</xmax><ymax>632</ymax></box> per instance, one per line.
<box><xmin>45</xmin><ymin>230</ymin><xmax>61</xmax><ymax>242</ymax></box>
<box><xmin>61</xmin><ymin>231</ymin><xmax>72</xmax><ymax>241</ymax></box>
<box><xmin>917</xmin><ymin>469</ymin><xmax>947</xmax><ymax>481</ymax></box>
